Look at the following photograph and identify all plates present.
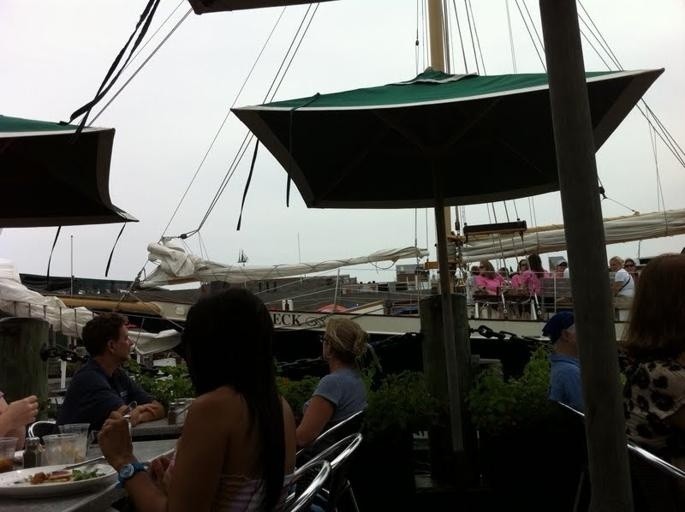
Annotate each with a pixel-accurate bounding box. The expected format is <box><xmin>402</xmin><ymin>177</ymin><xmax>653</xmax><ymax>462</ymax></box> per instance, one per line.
<box><xmin>0</xmin><ymin>462</ymin><xmax>116</xmax><ymax>497</ymax></box>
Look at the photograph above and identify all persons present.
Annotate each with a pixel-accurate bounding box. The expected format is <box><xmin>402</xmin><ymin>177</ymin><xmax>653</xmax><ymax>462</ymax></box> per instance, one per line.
<box><xmin>295</xmin><ymin>315</ymin><xmax>384</xmax><ymax>454</ymax></box>
<box><xmin>96</xmin><ymin>285</ymin><xmax>297</xmax><ymax>511</ymax></box>
<box><xmin>0</xmin><ymin>389</ymin><xmax>41</xmax><ymax>454</ymax></box>
<box><xmin>51</xmin><ymin>312</ymin><xmax>167</xmax><ymax>445</ymax></box>
<box><xmin>541</xmin><ymin>310</ymin><xmax>586</xmax><ymax>415</ymax></box>
<box><xmin>617</xmin><ymin>253</ymin><xmax>685</xmax><ymax>472</ymax></box>
<box><xmin>466</xmin><ymin>252</ymin><xmax>641</xmax><ymax>323</ymax></box>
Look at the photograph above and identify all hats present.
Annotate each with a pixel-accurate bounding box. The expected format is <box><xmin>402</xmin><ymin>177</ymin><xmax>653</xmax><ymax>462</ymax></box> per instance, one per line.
<box><xmin>541</xmin><ymin>310</ymin><xmax>574</xmax><ymax>344</ymax></box>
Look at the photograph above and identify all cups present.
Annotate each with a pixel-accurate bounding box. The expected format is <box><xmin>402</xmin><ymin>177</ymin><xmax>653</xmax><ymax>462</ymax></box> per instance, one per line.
<box><xmin>0</xmin><ymin>437</ymin><xmax>17</xmax><ymax>472</ymax></box>
<box><xmin>44</xmin><ymin>424</ymin><xmax>90</xmax><ymax>463</ymax></box>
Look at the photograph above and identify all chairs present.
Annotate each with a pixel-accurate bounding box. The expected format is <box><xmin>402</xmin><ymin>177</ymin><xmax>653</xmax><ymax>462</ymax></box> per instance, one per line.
<box><xmin>274</xmin><ymin>406</ymin><xmax>365</xmax><ymax>512</ymax></box>
<box><xmin>554</xmin><ymin>401</ymin><xmax>685</xmax><ymax>512</ymax></box>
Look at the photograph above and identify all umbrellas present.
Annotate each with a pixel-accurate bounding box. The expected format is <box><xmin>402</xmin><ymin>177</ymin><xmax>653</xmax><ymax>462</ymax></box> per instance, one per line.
<box><xmin>227</xmin><ymin>64</ymin><xmax>664</xmax><ymax>454</ymax></box>
<box><xmin>2</xmin><ymin>113</ymin><xmax>139</xmax><ymax>228</ymax></box>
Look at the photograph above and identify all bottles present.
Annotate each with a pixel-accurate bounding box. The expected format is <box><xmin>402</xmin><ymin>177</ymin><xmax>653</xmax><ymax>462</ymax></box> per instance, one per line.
<box><xmin>22</xmin><ymin>437</ymin><xmax>42</xmax><ymax>466</ymax></box>
<box><xmin>168</xmin><ymin>401</ymin><xmax>187</xmax><ymax>427</ymax></box>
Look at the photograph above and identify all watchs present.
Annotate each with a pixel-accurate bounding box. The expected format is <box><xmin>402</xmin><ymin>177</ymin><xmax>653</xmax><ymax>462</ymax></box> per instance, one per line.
<box><xmin>115</xmin><ymin>462</ymin><xmax>146</xmax><ymax>489</ymax></box>
